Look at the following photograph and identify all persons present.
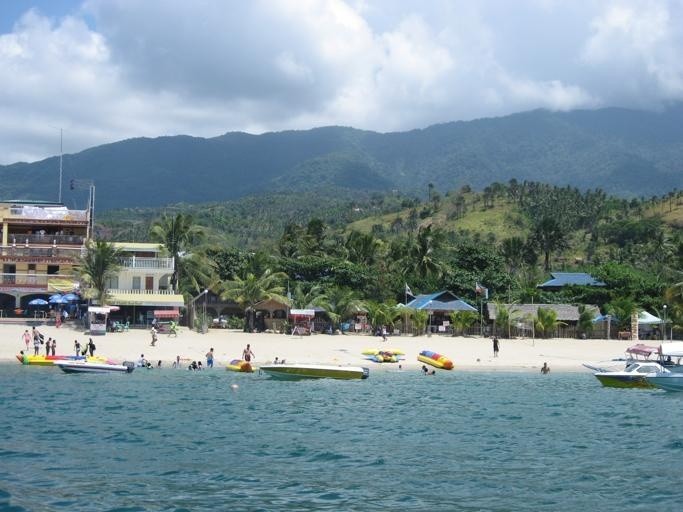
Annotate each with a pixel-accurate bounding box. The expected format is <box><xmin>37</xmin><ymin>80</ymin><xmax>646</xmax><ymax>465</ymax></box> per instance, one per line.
<box><xmin>56</xmin><ymin>312</ymin><xmax>61</xmax><ymax>328</ymax></box>
<box><xmin>382</xmin><ymin>326</ymin><xmax>387</xmax><ymax>340</ymax></box>
<box><xmin>9</xmin><ymin>229</ymin><xmax>80</xmax><ymax>243</ymax></box>
<box><xmin>398</xmin><ymin>365</ymin><xmax>435</xmax><ymax>376</ymax></box>
<box><xmin>540</xmin><ymin>363</ymin><xmax>550</xmax><ymax>374</ymax></box>
<box><xmin>492</xmin><ymin>338</ymin><xmax>501</xmax><ymax>357</ymax></box>
<box><xmin>21</xmin><ymin>318</ymin><xmax>285</xmax><ymax>370</ymax></box>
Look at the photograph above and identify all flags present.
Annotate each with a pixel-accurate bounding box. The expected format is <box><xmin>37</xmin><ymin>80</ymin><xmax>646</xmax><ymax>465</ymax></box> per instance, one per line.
<box><xmin>476</xmin><ymin>284</ymin><xmax>489</xmax><ymax>298</ymax></box>
<box><xmin>405</xmin><ymin>284</ymin><xmax>414</xmax><ymax>296</ymax></box>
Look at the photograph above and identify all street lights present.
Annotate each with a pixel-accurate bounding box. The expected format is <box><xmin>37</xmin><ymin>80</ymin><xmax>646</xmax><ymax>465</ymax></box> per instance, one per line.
<box><xmin>662</xmin><ymin>305</ymin><xmax>666</xmax><ymax>340</ymax></box>
<box><xmin>204</xmin><ymin>289</ymin><xmax>208</xmax><ymax>301</ymax></box>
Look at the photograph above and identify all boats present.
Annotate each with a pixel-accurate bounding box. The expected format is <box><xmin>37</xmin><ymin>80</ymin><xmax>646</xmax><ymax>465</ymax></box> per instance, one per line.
<box><xmin>52</xmin><ymin>360</ymin><xmax>134</xmax><ymax>373</ymax></box>
<box><xmin>416</xmin><ymin>349</ymin><xmax>453</xmax><ymax>370</ymax></box>
<box><xmin>15</xmin><ymin>354</ymin><xmax>119</xmax><ymax>365</ymax></box>
<box><xmin>259</xmin><ymin>364</ymin><xmax>369</xmax><ymax>380</ymax></box>
<box><xmin>225</xmin><ymin>359</ymin><xmax>256</xmax><ymax>373</ymax></box>
<box><xmin>581</xmin><ymin>343</ymin><xmax>683</xmax><ymax>373</ymax></box>
<box><xmin>591</xmin><ymin>361</ymin><xmax>682</xmax><ymax>391</ymax></box>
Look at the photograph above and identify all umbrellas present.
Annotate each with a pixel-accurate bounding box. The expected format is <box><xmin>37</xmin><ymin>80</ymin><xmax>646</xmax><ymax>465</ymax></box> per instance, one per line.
<box><xmin>28</xmin><ymin>293</ymin><xmax>79</xmax><ymax>313</ymax></box>
<box><xmin>591</xmin><ymin>313</ymin><xmax>619</xmax><ymax>339</ymax></box>
